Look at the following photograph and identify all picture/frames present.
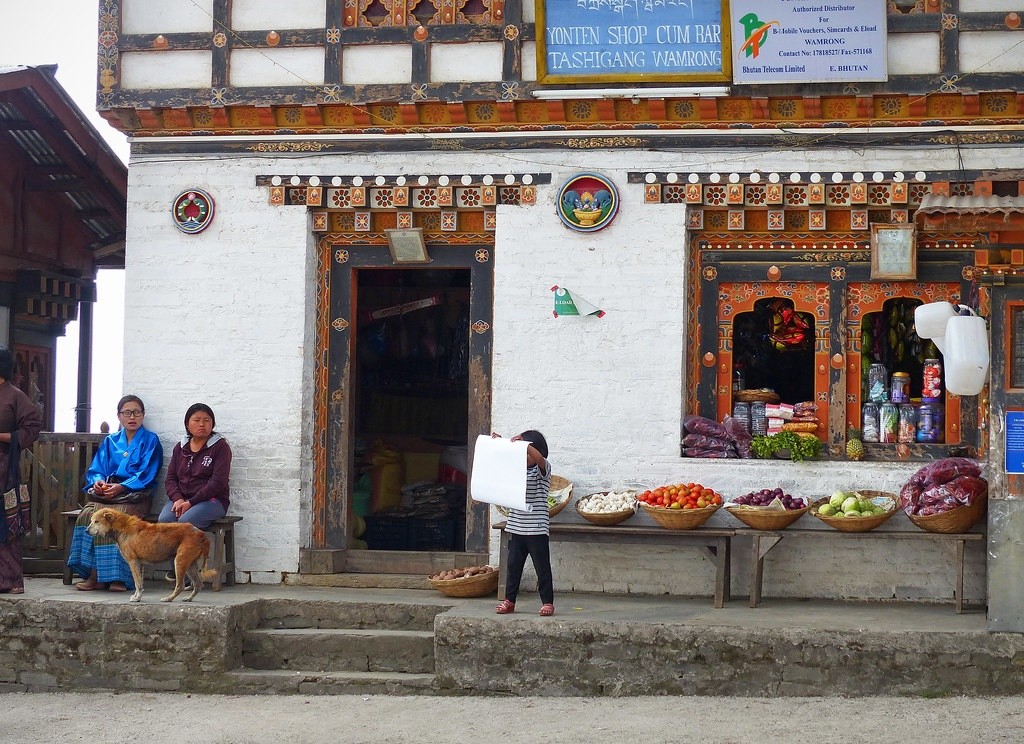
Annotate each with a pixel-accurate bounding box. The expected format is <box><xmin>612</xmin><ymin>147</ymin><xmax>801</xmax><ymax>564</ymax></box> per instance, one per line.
<box><xmin>869</xmin><ymin>223</ymin><xmax>917</xmax><ymax>281</ymax></box>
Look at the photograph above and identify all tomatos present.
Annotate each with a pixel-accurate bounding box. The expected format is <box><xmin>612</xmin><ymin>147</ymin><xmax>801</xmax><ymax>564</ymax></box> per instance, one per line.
<box><xmin>637</xmin><ymin>483</ymin><xmax>721</xmax><ymax>509</ymax></box>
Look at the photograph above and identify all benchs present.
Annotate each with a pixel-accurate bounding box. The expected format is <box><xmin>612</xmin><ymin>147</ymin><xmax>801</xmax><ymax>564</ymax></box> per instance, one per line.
<box><xmin>734</xmin><ymin>514</ymin><xmax>983</xmax><ymax>615</ymax></box>
<box><xmin>492</xmin><ymin>513</ymin><xmax>736</xmax><ymax>608</ymax></box>
<box><xmin>63</xmin><ymin>509</ymin><xmax>241</xmax><ymax>593</ymax></box>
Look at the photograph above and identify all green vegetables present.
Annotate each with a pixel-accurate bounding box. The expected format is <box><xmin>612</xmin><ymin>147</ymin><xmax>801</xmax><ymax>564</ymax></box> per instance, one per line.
<box><xmin>748</xmin><ymin>430</ymin><xmax>823</xmax><ymax>463</ymax></box>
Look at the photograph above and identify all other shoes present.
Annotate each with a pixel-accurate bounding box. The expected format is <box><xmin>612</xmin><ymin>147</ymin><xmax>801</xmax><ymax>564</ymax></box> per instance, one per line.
<box><xmin>7</xmin><ymin>586</ymin><xmax>24</xmax><ymax>593</ymax></box>
<box><xmin>539</xmin><ymin>604</ymin><xmax>553</xmax><ymax>616</ymax></box>
<box><xmin>184</xmin><ymin>582</ymin><xmax>192</xmax><ymax>589</ymax></box>
<box><xmin>165</xmin><ymin>571</ymin><xmax>176</xmax><ymax>581</ymax></box>
<box><xmin>496</xmin><ymin>600</ymin><xmax>514</xmax><ymax>614</ymax></box>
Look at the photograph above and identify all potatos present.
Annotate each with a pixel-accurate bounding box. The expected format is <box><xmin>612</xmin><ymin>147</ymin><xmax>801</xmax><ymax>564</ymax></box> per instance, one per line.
<box><xmin>433</xmin><ymin>566</ymin><xmax>493</xmax><ymax>581</ymax></box>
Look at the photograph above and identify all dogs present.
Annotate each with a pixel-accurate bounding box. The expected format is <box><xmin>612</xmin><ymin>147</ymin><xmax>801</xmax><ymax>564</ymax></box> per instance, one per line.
<box><xmin>85</xmin><ymin>507</ymin><xmax>210</xmax><ymax>602</ymax></box>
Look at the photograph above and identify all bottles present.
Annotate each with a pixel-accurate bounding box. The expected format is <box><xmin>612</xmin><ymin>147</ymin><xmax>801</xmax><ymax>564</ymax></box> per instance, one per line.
<box><xmin>751</xmin><ymin>400</ymin><xmax>768</xmax><ymax>436</ymax></box>
<box><xmin>733</xmin><ymin>401</ymin><xmax>750</xmax><ymax>434</ymax></box>
<box><xmin>897</xmin><ymin>404</ymin><xmax>917</xmax><ymax>442</ymax></box>
<box><xmin>732</xmin><ymin>363</ymin><xmax>745</xmax><ymax>392</ymax></box>
<box><xmin>862</xmin><ymin>403</ymin><xmax>879</xmax><ymax>442</ymax></box>
<box><xmin>891</xmin><ymin>372</ymin><xmax>910</xmax><ymax>403</ymax></box>
<box><xmin>916</xmin><ymin>399</ymin><xmax>945</xmax><ymax>444</ymax></box>
<box><xmin>879</xmin><ymin>403</ymin><xmax>898</xmax><ymax>442</ymax></box>
<box><xmin>922</xmin><ymin>359</ymin><xmax>942</xmax><ymax>399</ymax></box>
<box><xmin>868</xmin><ymin>364</ymin><xmax>887</xmax><ymax>404</ymax></box>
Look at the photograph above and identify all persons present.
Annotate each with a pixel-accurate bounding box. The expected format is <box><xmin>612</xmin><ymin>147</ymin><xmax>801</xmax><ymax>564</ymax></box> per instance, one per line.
<box><xmin>158</xmin><ymin>403</ymin><xmax>232</xmax><ymax>590</ymax></box>
<box><xmin>491</xmin><ymin>430</ymin><xmax>554</xmax><ymax>616</ymax></box>
<box><xmin>0</xmin><ymin>346</ymin><xmax>42</xmax><ymax>594</ymax></box>
<box><xmin>67</xmin><ymin>395</ymin><xmax>163</xmax><ymax>592</ymax></box>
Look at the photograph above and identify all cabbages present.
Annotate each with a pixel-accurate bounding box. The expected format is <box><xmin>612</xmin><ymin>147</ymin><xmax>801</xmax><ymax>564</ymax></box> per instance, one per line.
<box><xmin>817</xmin><ymin>491</ymin><xmax>883</xmax><ymax>518</ymax></box>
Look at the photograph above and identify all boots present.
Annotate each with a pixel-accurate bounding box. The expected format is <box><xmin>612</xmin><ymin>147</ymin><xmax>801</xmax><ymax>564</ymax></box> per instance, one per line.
<box><xmin>109</xmin><ymin>580</ymin><xmax>126</xmax><ymax>591</ymax></box>
<box><xmin>76</xmin><ymin>569</ymin><xmax>106</xmax><ymax>590</ymax></box>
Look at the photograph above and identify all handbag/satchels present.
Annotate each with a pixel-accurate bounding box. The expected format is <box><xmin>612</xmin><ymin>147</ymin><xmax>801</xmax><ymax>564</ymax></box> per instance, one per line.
<box><xmin>0</xmin><ymin>484</ymin><xmax>32</xmax><ymax>544</ymax></box>
<box><xmin>87</xmin><ymin>485</ymin><xmax>147</xmax><ymax>503</ymax></box>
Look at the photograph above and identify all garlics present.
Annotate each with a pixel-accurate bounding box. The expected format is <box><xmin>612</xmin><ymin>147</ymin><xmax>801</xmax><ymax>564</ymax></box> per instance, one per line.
<box><xmin>578</xmin><ymin>490</ymin><xmax>635</xmax><ymax>513</ymax></box>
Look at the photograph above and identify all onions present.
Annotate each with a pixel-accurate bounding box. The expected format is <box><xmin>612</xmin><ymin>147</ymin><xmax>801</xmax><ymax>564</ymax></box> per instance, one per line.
<box><xmin>733</xmin><ymin>488</ymin><xmax>806</xmax><ymax>510</ymax></box>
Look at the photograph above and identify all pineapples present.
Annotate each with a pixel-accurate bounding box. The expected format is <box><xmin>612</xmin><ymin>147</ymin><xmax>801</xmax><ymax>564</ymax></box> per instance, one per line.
<box><xmin>846</xmin><ymin>428</ymin><xmax>864</xmax><ymax>460</ymax></box>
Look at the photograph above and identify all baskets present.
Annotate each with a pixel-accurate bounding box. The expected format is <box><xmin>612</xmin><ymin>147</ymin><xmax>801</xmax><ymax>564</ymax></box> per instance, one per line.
<box><xmin>726</xmin><ymin>504</ymin><xmax>810</xmax><ymax>530</ymax></box>
<box><xmin>638</xmin><ymin>501</ymin><xmax>724</xmax><ymax>530</ymax></box>
<box><xmin>428</xmin><ymin>568</ymin><xmax>499</xmax><ymax>597</ymax></box>
<box><xmin>575</xmin><ymin>492</ymin><xmax>639</xmax><ymax>526</ymax></box>
<box><xmin>807</xmin><ymin>490</ymin><xmax>900</xmax><ymax>532</ymax></box>
<box><xmin>900</xmin><ymin>477</ymin><xmax>988</xmax><ymax>534</ymax></box>
<box><xmin>495</xmin><ymin>474</ymin><xmax>573</xmax><ymax>518</ymax></box>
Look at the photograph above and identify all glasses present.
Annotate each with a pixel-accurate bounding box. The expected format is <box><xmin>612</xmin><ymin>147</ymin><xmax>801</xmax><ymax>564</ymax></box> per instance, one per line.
<box><xmin>118</xmin><ymin>409</ymin><xmax>143</xmax><ymax>417</ymax></box>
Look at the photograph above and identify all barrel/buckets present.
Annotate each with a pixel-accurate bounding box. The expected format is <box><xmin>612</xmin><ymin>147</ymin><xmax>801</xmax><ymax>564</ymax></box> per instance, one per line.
<box><xmin>932</xmin><ymin>304</ymin><xmax>985</xmax><ymax>354</ymax></box>
<box><xmin>944</xmin><ymin>310</ymin><xmax>989</xmax><ymax>396</ymax></box>
<box><xmin>914</xmin><ymin>301</ymin><xmax>961</xmax><ymax>339</ymax></box>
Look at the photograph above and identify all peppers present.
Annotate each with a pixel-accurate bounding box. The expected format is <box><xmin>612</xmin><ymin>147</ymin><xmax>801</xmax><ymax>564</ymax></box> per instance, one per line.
<box><xmin>548</xmin><ymin>496</ymin><xmax>556</xmax><ymax>508</ymax></box>
